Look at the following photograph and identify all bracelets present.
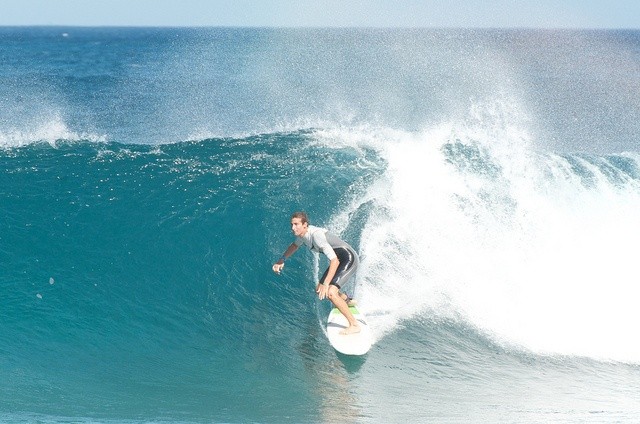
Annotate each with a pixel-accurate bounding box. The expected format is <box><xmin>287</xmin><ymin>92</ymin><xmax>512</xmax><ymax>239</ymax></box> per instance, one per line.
<box><xmin>322</xmin><ymin>282</ymin><xmax>329</xmax><ymax>287</ymax></box>
<box><xmin>281</xmin><ymin>255</ymin><xmax>287</xmax><ymax>262</ymax></box>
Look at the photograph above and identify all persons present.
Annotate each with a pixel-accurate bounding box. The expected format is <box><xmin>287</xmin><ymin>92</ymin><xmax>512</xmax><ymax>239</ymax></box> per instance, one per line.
<box><xmin>272</xmin><ymin>212</ymin><xmax>361</xmax><ymax>335</ymax></box>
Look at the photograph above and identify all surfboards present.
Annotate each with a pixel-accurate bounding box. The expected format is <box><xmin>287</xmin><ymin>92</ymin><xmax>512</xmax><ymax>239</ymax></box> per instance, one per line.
<box><xmin>327</xmin><ymin>305</ymin><xmax>371</xmax><ymax>356</ymax></box>
